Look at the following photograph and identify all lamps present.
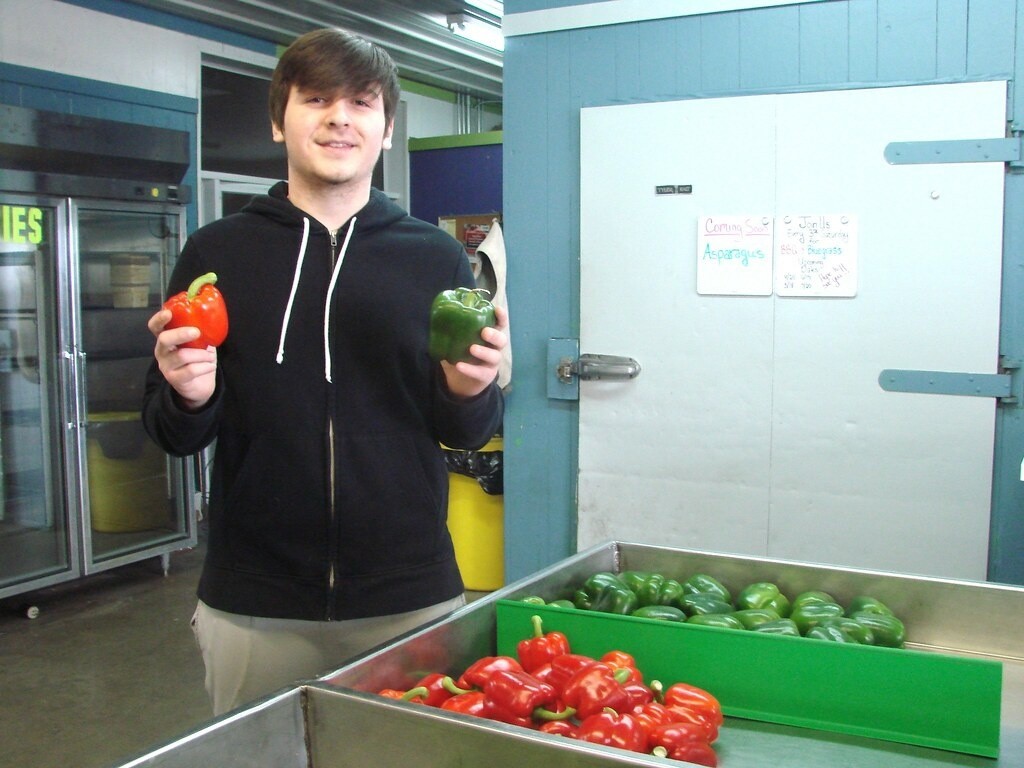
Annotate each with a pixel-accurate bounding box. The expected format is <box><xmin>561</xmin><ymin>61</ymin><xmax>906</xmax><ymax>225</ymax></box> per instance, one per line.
<box><xmin>447</xmin><ymin>15</ymin><xmax>504</xmax><ymax>52</ymax></box>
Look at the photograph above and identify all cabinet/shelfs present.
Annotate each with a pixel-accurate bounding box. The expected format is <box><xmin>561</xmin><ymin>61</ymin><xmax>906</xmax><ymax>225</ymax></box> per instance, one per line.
<box><xmin>0</xmin><ymin>190</ymin><xmax>199</xmax><ymax>604</ymax></box>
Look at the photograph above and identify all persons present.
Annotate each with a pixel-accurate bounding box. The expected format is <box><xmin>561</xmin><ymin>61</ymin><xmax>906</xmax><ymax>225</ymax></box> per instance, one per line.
<box><xmin>139</xmin><ymin>24</ymin><xmax>509</xmax><ymax>716</ymax></box>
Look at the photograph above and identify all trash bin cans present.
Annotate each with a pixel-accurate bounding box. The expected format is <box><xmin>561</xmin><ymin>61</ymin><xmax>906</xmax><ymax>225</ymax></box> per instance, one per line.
<box><xmin>83</xmin><ymin>412</ymin><xmax>172</xmax><ymax>532</ymax></box>
<box><xmin>433</xmin><ymin>435</ymin><xmax>507</xmax><ymax>591</ymax></box>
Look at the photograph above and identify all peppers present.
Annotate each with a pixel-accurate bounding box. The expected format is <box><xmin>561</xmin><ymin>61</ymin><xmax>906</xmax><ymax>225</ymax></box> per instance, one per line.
<box><xmin>379</xmin><ymin>569</ymin><xmax>904</xmax><ymax>767</ymax></box>
<box><xmin>163</xmin><ymin>272</ymin><xmax>228</xmax><ymax>350</ymax></box>
<box><xmin>429</xmin><ymin>287</ymin><xmax>497</xmax><ymax>367</ymax></box>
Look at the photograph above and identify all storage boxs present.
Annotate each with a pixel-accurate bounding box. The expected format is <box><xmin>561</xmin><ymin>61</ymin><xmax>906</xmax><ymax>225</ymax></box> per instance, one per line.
<box><xmin>114</xmin><ymin>540</ymin><xmax>1024</xmax><ymax>767</ymax></box>
